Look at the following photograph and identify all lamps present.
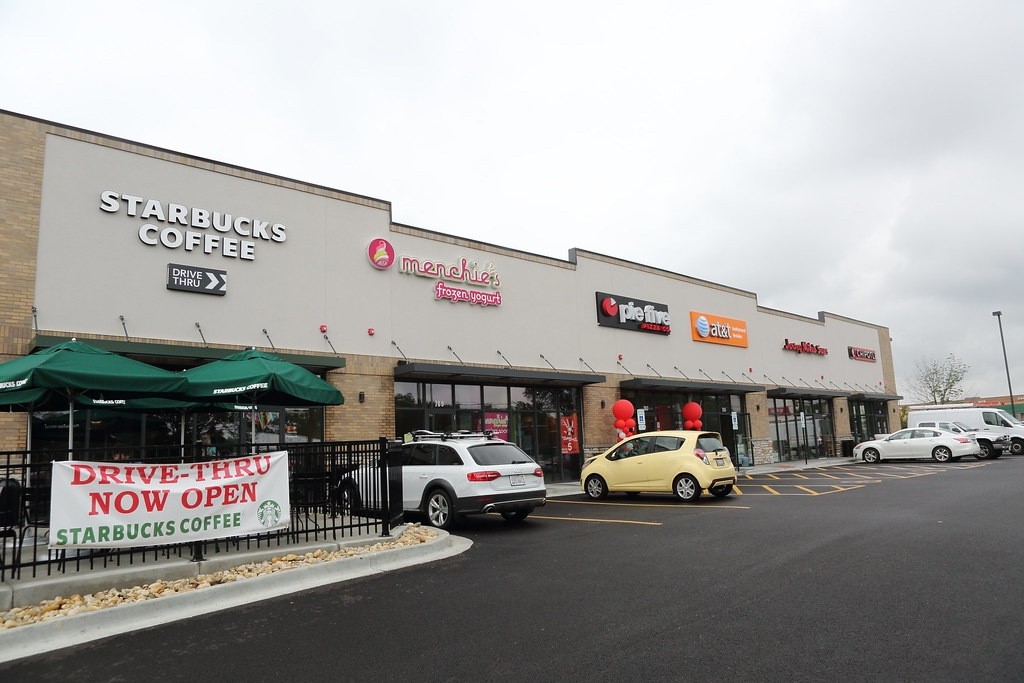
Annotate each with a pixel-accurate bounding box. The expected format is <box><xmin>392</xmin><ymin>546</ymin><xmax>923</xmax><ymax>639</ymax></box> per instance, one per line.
<box><xmin>359</xmin><ymin>392</ymin><xmax>364</xmax><ymax>402</ymax></box>
<box><xmin>601</xmin><ymin>400</ymin><xmax>606</xmax><ymax>408</ymax></box>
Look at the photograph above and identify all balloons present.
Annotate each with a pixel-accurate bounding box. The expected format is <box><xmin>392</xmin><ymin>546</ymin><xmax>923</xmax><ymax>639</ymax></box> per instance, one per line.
<box><xmin>694</xmin><ymin>419</ymin><xmax>702</xmax><ymax>428</ymax></box>
<box><xmin>614</xmin><ymin>420</ymin><xmax>618</xmax><ymax>429</ymax></box>
<box><xmin>613</xmin><ymin>400</ymin><xmax>634</xmax><ymax>421</ymax></box>
<box><xmin>626</xmin><ymin>419</ymin><xmax>635</xmax><ymax>428</ymax></box>
<box><xmin>684</xmin><ymin>420</ymin><xmax>693</xmax><ymax>430</ymax></box>
<box><xmin>622</xmin><ymin>427</ymin><xmax>637</xmax><ymax>437</ymax></box>
<box><xmin>617</xmin><ymin>428</ymin><xmax>622</xmax><ymax>433</ymax></box>
<box><xmin>622</xmin><ymin>442</ymin><xmax>633</xmax><ymax>451</ymax></box>
<box><xmin>617</xmin><ymin>438</ymin><xmax>623</xmax><ymax>443</ymax></box>
<box><xmin>619</xmin><ymin>432</ymin><xmax>626</xmax><ymax>439</ymax></box>
<box><xmin>683</xmin><ymin>402</ymin><xmax>702</xmax><ymax>421</ymax></box>
<box><xmin>615</xmin><ymin>420</ymin><xmax>625</xmax><ymax>428</ymax></box>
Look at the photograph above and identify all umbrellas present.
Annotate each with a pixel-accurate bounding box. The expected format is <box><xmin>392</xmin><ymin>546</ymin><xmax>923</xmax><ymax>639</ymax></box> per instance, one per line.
<box><xmin>0</xmin><ymin>338</ymin><xmax>193</xmax><ymax>460</ymax></box>
<box><xmin>32</xmin><ymin>397</ymin><xmax>236</xmax><ymax>462</ymax></box>
<box><xmin>181</xmin><ymin>349</ymin><xmax>345</xmax><ymax>455</ymax></box>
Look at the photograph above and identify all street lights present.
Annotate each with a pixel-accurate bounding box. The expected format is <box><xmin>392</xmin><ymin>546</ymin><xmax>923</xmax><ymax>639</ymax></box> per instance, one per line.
<box><xmin>992</xmin><ymin>310</ymin><xmax>1016</xmax><ymax>418</ymax></box>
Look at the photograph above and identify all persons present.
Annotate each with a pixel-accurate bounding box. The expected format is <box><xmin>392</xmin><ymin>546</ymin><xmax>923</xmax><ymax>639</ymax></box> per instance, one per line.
<box><xmin>112</xmin><ymin>443</ymin><xmax>130</xmax><ymax>460</ymax></box>
<box><xmin>202</xmin><ymin>432</ymin><xmax>220</xmax><ymax>457</ymax></box>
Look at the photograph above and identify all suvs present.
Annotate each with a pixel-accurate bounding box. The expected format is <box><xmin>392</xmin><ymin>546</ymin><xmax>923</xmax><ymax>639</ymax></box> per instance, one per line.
<box><xmin>335</xmin><ymin>429</ymin><xmax>547</xmax><ymax>530</ymax></box>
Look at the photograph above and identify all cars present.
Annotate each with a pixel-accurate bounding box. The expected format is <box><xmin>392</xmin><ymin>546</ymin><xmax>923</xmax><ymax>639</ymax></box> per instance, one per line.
<box><xmin>250</xmin><ymin>432</ymin><xmax>322</xmax><ymax>476</ymax></box>
<box><xmin>852</xmin><ymin>427</ymin><xmax>981</xmax><ymax>462</ymax></box>
<box><xmin>580</xmin><ymin>430</ymin><xmax>738</xmax><ymax>501</ymax></box>
<box><xmin>529</xmin><ymin>444</ymin><xmax>578</xmax><ymax>473</ymax></box>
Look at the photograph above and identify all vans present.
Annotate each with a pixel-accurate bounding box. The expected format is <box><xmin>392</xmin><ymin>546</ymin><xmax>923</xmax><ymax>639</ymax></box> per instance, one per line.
<box><xmin>908</xmin><ymin>406</ymin><xmax>1024</xmax><ymax>455</ymax></box>
<box><xmin>770</xmin><ymin>415</ymin><xmax>823</xmax><ymax>456</ymax></box>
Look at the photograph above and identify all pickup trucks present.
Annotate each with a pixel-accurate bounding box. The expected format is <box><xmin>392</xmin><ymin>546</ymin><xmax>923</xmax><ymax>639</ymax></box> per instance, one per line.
<box><xmin>873</xmin><ymin>421</ymin><xmax>1014</xmax><ymax>460</ymax></box>
